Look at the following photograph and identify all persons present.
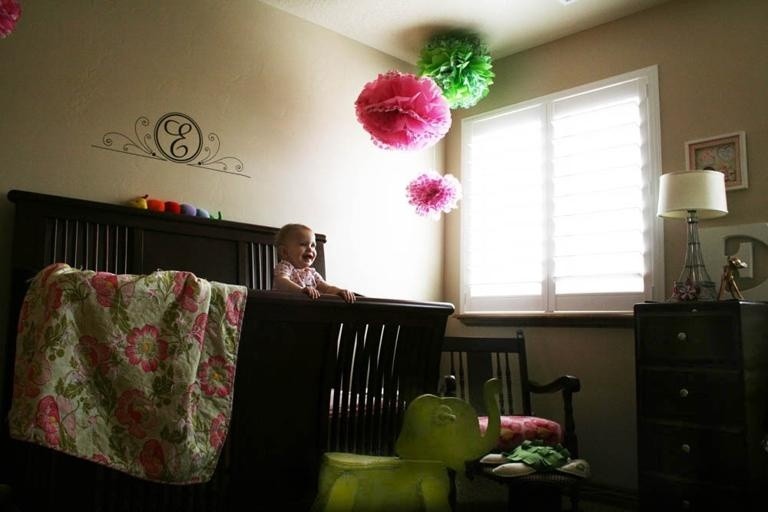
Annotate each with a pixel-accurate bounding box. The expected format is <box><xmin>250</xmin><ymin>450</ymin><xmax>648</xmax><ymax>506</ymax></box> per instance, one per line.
<box><xmin>272</xmin><ymin>222</ymin><xmax>358</xmax><ymax>305</ymax></box>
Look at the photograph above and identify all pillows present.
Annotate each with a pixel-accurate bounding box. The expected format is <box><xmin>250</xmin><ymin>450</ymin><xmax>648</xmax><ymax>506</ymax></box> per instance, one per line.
<box><xmin>479</xmin><ymin>416</ymin><xmax>563</xmax><ymax>448</ymax></box>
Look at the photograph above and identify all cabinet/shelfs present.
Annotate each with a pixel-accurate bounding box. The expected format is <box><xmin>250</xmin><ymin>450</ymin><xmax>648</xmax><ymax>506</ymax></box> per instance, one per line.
<box><xmin>633</xmin><ymin>298</ymin><xmax>768</xmax><ymax>512</ymax></box>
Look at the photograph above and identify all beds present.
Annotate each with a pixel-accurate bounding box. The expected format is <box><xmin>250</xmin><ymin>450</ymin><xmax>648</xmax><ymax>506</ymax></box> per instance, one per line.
<box><xmin>1</xmin><ymin>187</ymin><xmax>456</xmax><ymax>512</ymax></box>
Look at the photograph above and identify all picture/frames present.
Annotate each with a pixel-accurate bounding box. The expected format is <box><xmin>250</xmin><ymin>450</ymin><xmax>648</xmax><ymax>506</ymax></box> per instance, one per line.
<box><xmin>684</xmin><ymin>131</ymin><xmax>749</xmax><ymax>191</ymax></box>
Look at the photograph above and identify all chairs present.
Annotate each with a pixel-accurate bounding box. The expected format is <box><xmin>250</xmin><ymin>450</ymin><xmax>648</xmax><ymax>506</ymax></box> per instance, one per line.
<box><xmin>441</xmin><ymin>329</ymin><xmax>582</xmax><ymax>512</ymax></box>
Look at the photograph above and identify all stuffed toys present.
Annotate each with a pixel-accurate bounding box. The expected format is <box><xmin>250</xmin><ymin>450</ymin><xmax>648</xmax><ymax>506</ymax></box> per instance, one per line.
<box><xmin>479</xmin><ymin>437</ymin><xmax>592</xmax><ymax>479</ymax></box>
<box><xmin>125</xmin><ymin>194</ymin><xmax>225</xmax><ymax>223</ymax></box>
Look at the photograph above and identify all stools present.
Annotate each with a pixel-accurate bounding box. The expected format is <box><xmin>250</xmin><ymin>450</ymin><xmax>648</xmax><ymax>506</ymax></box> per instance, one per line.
<box><xmin>466</xmin><ymin>456</ymin><xmax>581</xmax><ymax>512</ymax></box>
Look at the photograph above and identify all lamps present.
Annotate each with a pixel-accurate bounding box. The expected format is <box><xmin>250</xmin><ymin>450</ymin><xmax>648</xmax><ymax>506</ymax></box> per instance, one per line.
<box><xmin>656</xmin><ymin>170</ymin><xmax>728</xmax><ymax>303</ymax></box>
